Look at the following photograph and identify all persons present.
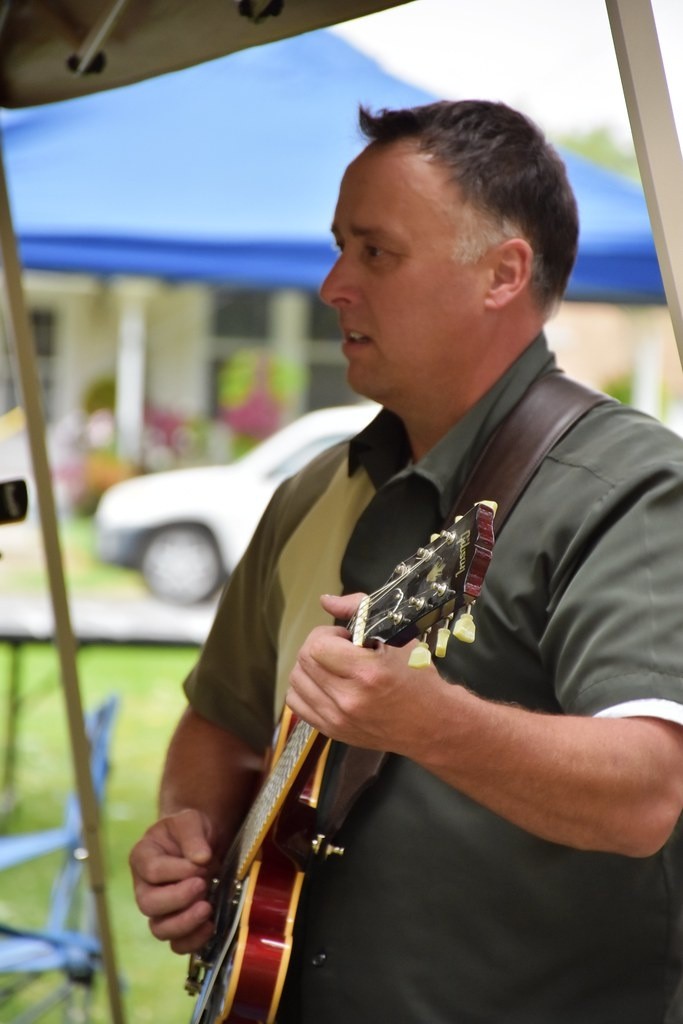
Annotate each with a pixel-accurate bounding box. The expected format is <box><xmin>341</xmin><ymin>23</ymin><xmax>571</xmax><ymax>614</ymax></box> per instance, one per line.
<box><xmin>128</xmin><ymin>97</ymin><xmax>682</xmax><ymax>1024</ymax></box>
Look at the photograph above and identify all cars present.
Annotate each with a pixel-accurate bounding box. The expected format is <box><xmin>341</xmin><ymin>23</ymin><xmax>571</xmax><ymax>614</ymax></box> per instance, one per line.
<box><xmin>82</xmin><ymin>398</ymin><xmax>387</xmax><ymax>610</ymax></box>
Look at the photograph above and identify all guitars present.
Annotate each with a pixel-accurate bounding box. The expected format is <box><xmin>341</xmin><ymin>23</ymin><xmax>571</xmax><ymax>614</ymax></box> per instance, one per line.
<box><xmin>184</xmin><ymin>499</ymin><xmax>499</xmax><ymax>1024</ymax></box>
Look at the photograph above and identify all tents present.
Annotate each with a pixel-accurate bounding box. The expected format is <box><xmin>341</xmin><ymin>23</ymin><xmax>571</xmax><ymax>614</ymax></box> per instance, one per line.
<box><xmin>0</xmin><ymin>28</ymin><xmax>673</xmax><ymax>465</ymax></box>
